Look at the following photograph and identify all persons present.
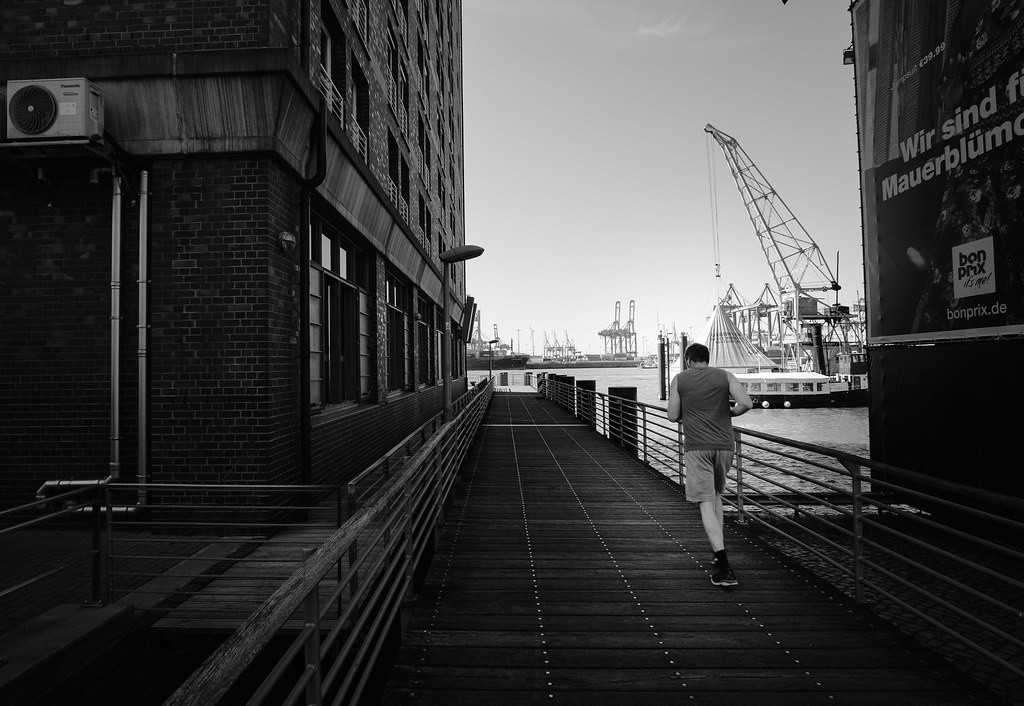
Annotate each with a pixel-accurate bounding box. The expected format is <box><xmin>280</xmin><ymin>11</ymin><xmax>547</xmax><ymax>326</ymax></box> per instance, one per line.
<box><xmin>667</xmin><ymin>343</ymin><xmax>753</xmax><ymax>586</ymax></box>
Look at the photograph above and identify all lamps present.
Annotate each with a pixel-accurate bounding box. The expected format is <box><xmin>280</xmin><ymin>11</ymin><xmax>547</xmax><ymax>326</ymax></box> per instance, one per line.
<box><xmin>843</xmin><ymin>44</ymin><xmax>854</xmax><ymax>65</ymax></box>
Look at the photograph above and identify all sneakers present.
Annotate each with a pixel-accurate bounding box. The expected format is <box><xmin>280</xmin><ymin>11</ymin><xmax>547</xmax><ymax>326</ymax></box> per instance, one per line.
<box><xmin>711</xmin><ymin>568</ymin><xmax>738</xmax><ymax>586</ymax></box>
<box><xmin>710</xmin><ymin>555</ymin><xmax>719</xmax><ymax>564</ymax></box>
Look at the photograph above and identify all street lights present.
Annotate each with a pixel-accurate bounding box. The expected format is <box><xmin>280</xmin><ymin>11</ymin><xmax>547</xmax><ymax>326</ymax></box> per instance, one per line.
<box><xmin>488</xmin><ymin>340</ymin><xmax>499</xmax><ymax>370</ymax></box>
<box><xmin>439</xmin><ymin>245</ymin><xmax>484</xmax><ymax>423</ymax></box>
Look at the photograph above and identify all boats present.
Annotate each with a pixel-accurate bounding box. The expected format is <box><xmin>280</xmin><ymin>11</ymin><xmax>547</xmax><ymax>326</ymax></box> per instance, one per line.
<box><xmin>466</xmin><ymin>310</ymin><xmax>530</xmax><ymax>370</ymax></box>
<box><xmin>642</xmin><ymin>365</ymin><xmax>658</xmax><ymax>369</ymax></box>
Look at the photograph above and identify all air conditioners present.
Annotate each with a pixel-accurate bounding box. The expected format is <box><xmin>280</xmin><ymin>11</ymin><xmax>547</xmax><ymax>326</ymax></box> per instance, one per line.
<box><xmin>4</xmin><ymin>78</ymin><xmax>104</xmax><ymax>142</ymax></box>
